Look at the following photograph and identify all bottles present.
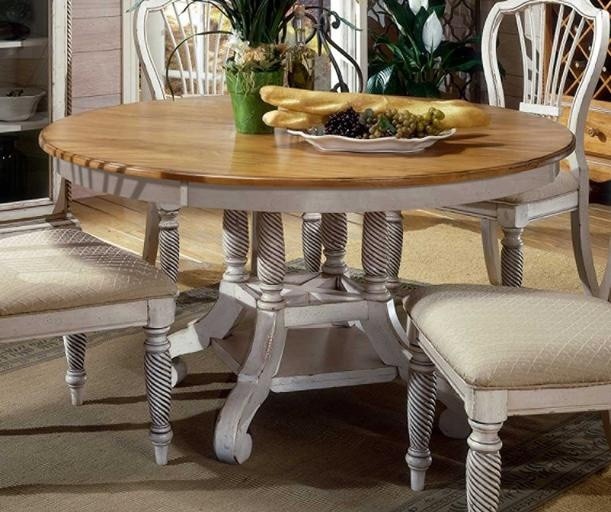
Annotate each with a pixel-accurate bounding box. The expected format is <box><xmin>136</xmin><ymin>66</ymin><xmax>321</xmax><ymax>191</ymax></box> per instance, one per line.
<box><xmin>1</xmin><ymin>137</ymin><xmax>24</xmax><ymax>201</ymax></box>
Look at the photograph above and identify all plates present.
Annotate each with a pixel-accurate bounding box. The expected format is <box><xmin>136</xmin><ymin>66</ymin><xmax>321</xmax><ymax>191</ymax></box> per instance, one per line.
<box><xmin>1</xmin><ymin>20</ymin><xmax>30</xmax><ymax>41</ymax></box>
<box><xmin>286</xmin><ymin>127</ymin><xmax>457</xmax><ymax>153</ymax></box>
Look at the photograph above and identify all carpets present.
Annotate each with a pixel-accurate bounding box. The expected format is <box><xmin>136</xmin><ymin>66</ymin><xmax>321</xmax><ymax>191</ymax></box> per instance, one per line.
<box><xmin>0</xmin><ymin>258</ymin><xmax>610</xmax><ymax>512</ymax></box>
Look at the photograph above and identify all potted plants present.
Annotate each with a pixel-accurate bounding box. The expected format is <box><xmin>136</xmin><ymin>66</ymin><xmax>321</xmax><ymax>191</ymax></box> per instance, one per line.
<box><xmin>163</xmin><ymin>0</ymin><xmax>364</xmax><ymax>135</ymax></box>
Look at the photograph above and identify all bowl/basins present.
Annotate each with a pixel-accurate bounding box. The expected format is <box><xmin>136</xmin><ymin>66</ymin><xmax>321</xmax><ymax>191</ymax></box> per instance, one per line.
<box><xmin>1</xmin><ymin>83</ymin><xmax>47</xmax><ymax>121</ymax></box>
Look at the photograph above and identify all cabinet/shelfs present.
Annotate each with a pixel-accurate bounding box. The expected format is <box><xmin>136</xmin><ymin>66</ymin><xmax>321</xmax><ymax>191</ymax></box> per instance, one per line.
<box><xmin>0</xmin><ymin>0</ymin><xmax>68</xmax><ymax>236</ymax></box>
<box><xmin>540</xmin><ymin>0</ymin><xmax>611</xmax><ymax>184</ymax></box>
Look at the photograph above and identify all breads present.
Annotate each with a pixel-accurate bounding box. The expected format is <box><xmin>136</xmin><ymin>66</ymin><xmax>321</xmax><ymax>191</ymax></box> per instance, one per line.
<box><xmin>254</xmin><ymin>83</ymin><xmax>492</xmax><ymax>129</ymax></box>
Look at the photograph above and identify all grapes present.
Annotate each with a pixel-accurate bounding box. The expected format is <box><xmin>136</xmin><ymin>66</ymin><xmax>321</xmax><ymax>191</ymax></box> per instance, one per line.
<box><xmin>323</xmin><ymin>107</ymin><xmax>446</xmax><ymax>140</ymax></box>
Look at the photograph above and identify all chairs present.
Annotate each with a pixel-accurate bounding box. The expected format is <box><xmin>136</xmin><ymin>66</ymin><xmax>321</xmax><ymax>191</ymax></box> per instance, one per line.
<box><xmin>406</xmin><ymin>245</ymin><xmax>611</xmax><ymax>512</ymax></box>
<box><xmin>384</xmin><ymin>0</ymin><xmax>610</xmax><ymax>298</ymax></box>
<box><xmin>0</xmin><ymin>228</ymin><xmax>173</xmax><ymax>467</ymax></box>
<box><xmin>134</xmin><ymin>0</ymin><xmax>323</xmax><ymax>276</ymax></box>
